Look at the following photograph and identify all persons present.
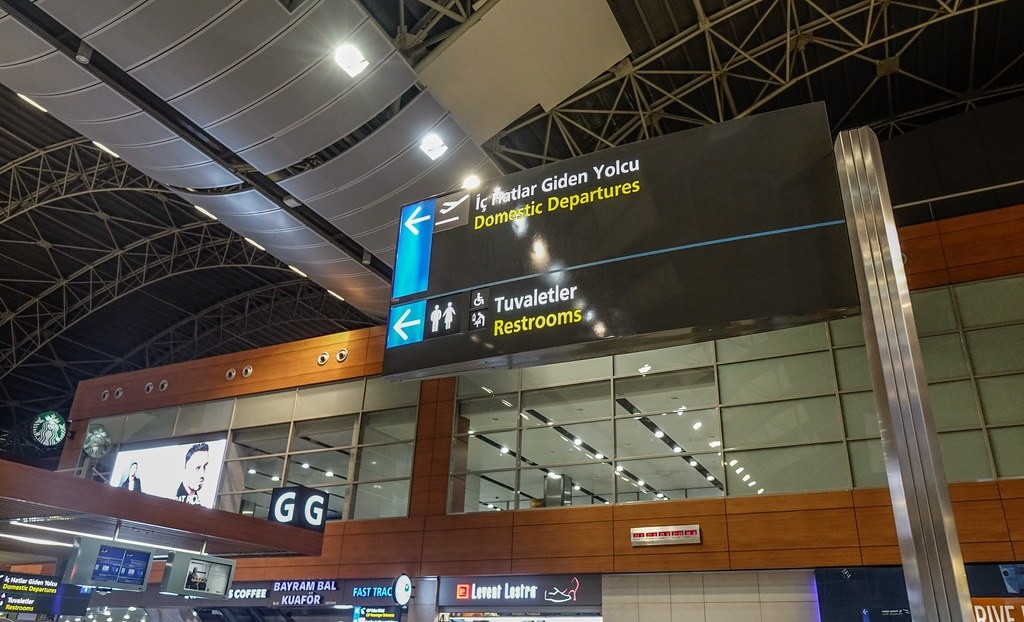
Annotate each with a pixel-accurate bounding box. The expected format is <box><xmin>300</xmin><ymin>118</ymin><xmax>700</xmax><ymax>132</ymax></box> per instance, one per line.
<box><xmin>188</xmin><ymin>567</ymin><xmax>198</xmax><ymax>589</ymax></box>
<box><xmin>36</xmin><ymin>414</ymin><xmax>60</xmax><ymax>445</ymax></box>
<box><xmin>121</xmin><ymin>462</ymin><xmax>141</xmax><ymax>492</ymax></box>
<box><xmin>84</xmin><ymin>429</ymin><xmax>108</xmax><ymax>458</ymax></box>
<box><xmin>176</xmin><ymin>444</ymin><xmax>209</xmax><ymax>506</ymax></box>
